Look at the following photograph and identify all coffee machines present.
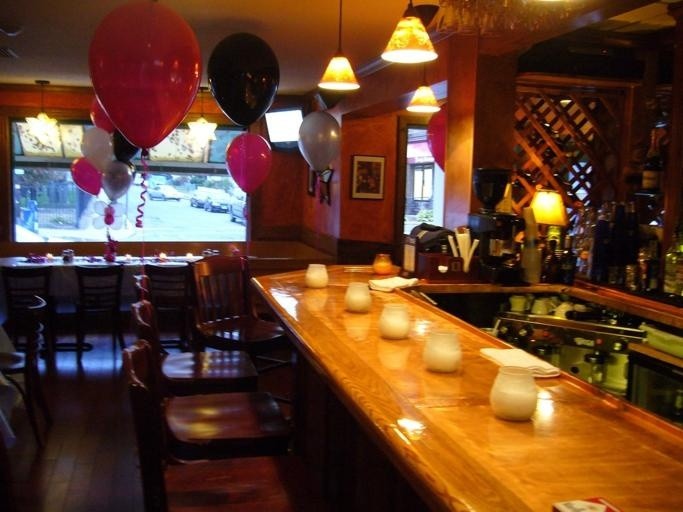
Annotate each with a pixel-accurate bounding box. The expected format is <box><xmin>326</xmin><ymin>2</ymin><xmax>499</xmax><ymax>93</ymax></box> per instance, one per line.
<box><xmin>492</xmin><ymin>312</ymin><xmax>645</xmax><ymax>399</ymax></box>
<box><xmin>466</xmin><ymin>166</ymin><xmax>525</xmax><ymax>284</ymax></box>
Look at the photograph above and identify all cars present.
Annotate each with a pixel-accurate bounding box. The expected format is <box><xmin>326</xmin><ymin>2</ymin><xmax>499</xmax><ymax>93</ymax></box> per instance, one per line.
<box><xmin>133</xmin><ymin>174</ymin><xmax>247</xmax><ymax>223</ymax></box>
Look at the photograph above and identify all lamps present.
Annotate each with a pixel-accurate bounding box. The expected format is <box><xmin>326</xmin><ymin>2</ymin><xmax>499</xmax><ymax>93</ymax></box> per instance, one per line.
<box><xmin>530</xmin><ymin>189</ymin><xmax>570</xmax><ymax>269</ymax></box>
<box><xmin>380</xmin><ymin>0</ymin><xmax>439</xmax><ymax>63</ymax></box>
<box><xmin>317</xmin><ymin>0</ymin><xmax>360</xmax><ymax>90</ymax></box>
<box><xmin>406</xmin><ymin>4</ymin><xmax>442</xmax><ymax>113</ymax></box>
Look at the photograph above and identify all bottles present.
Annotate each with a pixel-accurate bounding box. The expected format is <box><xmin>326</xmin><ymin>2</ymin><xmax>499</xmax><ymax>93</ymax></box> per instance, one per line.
<box><xmin>544</xmin><ymin>200</ymin><xmax>683</xmax><ymax>299</ymax></box>
<box><xmin>643</xmin><ymin>129</ymin><xmax>661</xmax><ymax>191</ymax></box>
<box><xmin>203</xmin><ymin>249</ymin><xmax>220</xmax><ymax>259</ymax></box>
<box><xmin>305</xmin><ymin>254</ymin><xmax>539</xmax><ymax>423</ymax></box>
<box><xmin>63</xmin><ymin>249</ymin><xmax>73</xmax><ymax>262</ymax></box>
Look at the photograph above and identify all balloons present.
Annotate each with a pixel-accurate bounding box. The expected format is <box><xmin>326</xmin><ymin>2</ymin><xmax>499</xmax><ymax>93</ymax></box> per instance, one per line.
<box><xmin>225</xmin><ymin>131</ymin><xmax>274</xmax><ymax>194</ymax></box>
<box><xmin>99</xmin><ymin>159</ymin><xmax>132</xmax><ymax>201</ymax></box>
<box><xmin>110</xmin><ymin>128</ymin><xmax>138</xmax><ymax>161</ymax></box>
<box><xmin>205</xmin><ymin>28</ymin><xmax>281</xmax><ymax>127</ymax></box>
<box><xmin>86</xmin><ymin>1</ymin><xmax>202</xmax><ymax>155</ymax></box>
<box><xmin>77</xmin><ymin>127</ymin><xmax>110</xmax><ymax>174</ymax></box>
<box><xmin>422</xmin><ymin>103</ymin><xmax>446</xmax><ymax>173</ymax></box>
<box><xmin>90</xmin><ymin>201</ymin><xmax>125</xmax><ymax>231</ymax></box>
<box><xmin>296</xmin><ymin>110</ymin><xmax>342</xmax><ymax>173</ymax></box>
<box><xmin>88</xmin><ymin>96</ymin><xmax>116</xmax><ymax>135</ymax></box>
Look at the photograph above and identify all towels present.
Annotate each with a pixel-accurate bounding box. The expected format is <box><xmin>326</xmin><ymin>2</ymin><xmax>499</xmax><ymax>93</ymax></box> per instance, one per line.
<box><xmin>368</xmin><ymin>276</ymin><xmax>418</xmax><ymax>292</ymax></box>
<box><xmin>480</xmin><ymin>348</ymin><xmax>561</xmax><ymax>377</ymax></box>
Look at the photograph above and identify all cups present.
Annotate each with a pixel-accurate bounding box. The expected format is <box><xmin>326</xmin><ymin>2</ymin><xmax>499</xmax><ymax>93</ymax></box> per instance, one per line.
<box><xmin>509</xmin><ymin>295</ymin><xmax>586</xmax><ymax>319</ymax></box>
<box><xmin>477</xmin><ymin>326</ymin><xmax>498</xmax><ymax>338</ymax></box>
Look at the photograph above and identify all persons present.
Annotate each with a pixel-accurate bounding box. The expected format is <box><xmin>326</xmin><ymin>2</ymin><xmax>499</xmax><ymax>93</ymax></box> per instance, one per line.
<box><xmin>69</xmin><ymin>156</ymin><xmax>100</xmax><ymax>198</ymax></box>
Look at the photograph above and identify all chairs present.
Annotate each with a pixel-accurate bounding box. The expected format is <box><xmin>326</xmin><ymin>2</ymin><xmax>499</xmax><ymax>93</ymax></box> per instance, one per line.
<box><xmin>0</xmin><ymin>291</ymin><xmax>54</xmax><ymax>453</ymax></box>
<box><xmin>0</xmin><ymin>265</ymin><xmax>192</xmax><ymax>354</ymax></box>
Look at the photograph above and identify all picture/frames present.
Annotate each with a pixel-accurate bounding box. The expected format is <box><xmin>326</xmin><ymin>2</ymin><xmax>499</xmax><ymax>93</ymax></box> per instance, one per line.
<box><xmin>350</xmin><ymin>154</ymin><xmax>385</xmax><ymax>201</ymax></box>
